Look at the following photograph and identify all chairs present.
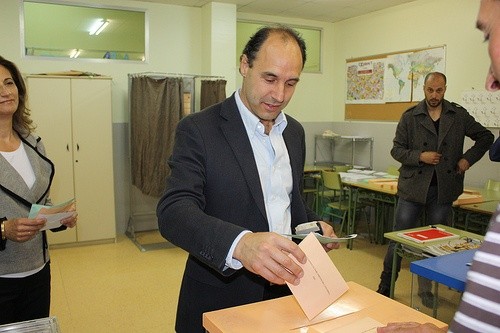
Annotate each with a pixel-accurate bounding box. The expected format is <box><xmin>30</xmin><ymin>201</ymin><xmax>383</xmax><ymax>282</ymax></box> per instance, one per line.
<box><xmin>314</xmin><ymin>169</ymin><xmax>372</xmax><ymax>251</ymax></box>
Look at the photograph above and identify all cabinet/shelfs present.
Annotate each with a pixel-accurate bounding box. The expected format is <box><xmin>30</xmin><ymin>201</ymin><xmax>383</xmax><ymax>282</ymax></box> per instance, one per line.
<box><xmin>25</xmin><ymin>75</ymin><xmax>118</xmax><ymax>251</ymax></box>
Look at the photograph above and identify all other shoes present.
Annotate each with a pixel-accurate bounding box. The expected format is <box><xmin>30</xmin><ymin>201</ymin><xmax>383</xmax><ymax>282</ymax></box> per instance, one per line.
<box><xmin>417</xmin><ymin>291</ymin><xmax>439</xmax><ymax>309</ymax></box>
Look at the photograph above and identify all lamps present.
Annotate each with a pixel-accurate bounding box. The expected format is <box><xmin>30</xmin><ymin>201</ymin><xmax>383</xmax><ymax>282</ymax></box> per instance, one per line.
<box><xmin>89</xmin><ymin>19</ymin><xmax>110</xmax><ymax>36</ymax></box>
<box><xmin>69</xmin><ymin>48</ymin><xmax>80</xmax><ymax>59</ymax></box>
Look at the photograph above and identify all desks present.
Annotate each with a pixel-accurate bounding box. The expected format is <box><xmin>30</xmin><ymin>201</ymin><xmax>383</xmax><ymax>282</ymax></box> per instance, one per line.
<box><xmin>303</xmin><ymin>162</ymin><xmax>369</xmax><ymax>251</ymax></box>
<box><xmin>459</xmin><ymin>200</ymin><xmax>500</xmax><ymax>236</ymax></box>
<box><xmin>382</xmin><ymin>223</ymin><xmax>485</xmax><ymax>319</ymax></box>
<box><xmin>410</xmin><ymin>247</ymin><xmax>478</xmax><ymax>319</ymax></box>
<box><xmin>350</xmin><ymin>180</ymin><xmax>484</xmax><ymax>246</ymax></box>
<box><xmin>314</xmin><ymin>134</ymin><xmax>374</xmax><ymax>171</ymax></box>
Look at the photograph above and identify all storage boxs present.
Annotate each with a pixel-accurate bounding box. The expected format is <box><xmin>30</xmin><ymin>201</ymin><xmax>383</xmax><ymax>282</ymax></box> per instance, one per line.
<box><xmin>201</xmin><ymin>278</ymin><xmax>449</xmax><ymax>333</ymax></box>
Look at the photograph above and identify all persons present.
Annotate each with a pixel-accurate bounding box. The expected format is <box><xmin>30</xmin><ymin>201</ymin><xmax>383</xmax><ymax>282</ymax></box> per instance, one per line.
<box><xmin>375</xmin><ymin>72</ymin><xmax>494</xmax><ymax>309</ymax></box>
<box><xmin>156</xmin><ymin>24</ymin><xmax>340</xmax><ymax>333</ymax></box>
<box><xmin>375</xmin><ymin>0</ymin><xmax>500</xmax><ymax>333</ymax></box>
<box><xmin>0</xmin><ymin>54</ymin><xmax>77</xmax><ymax>325</ymax></box>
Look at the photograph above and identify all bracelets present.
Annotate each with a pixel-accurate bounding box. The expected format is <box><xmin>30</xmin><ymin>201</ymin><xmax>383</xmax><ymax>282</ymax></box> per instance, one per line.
<box><xmin>0</xmin><ymin>220</ymin><xmax>5</xmax><ymax>240</ymax></box>
